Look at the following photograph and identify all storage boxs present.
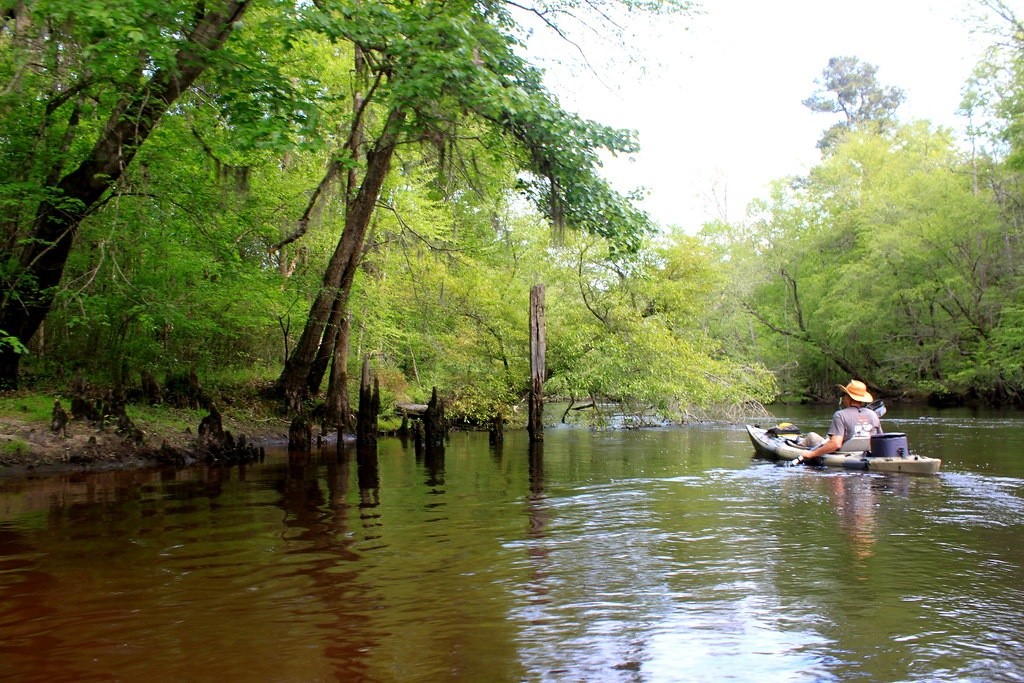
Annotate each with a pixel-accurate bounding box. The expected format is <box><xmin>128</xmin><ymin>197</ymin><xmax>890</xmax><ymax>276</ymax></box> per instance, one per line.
<box><xmin>870</xmin><ymin>431</ymin><xmax>908</xmax><ymax>457</ymax></box>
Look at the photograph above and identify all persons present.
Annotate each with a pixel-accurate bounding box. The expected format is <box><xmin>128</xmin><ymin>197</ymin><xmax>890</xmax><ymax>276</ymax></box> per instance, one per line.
<box><xmin>796</xmin><ymin>380</ymin><xmax>883</xmax><ymax>459</ymax></box>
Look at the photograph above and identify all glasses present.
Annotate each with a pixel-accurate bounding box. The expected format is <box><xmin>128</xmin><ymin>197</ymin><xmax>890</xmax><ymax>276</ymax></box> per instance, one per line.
<box><xmin>840</xmin><ymin>392</ymin><xmax>849</xmax><ymax>397</ymax></box>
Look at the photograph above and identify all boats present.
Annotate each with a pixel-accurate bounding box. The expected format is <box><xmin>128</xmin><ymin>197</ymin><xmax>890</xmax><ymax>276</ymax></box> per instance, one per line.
<box><xmin>745</xmin><ymin>425</ymin><xmax>941</xmax><ymax>475</ymax></box>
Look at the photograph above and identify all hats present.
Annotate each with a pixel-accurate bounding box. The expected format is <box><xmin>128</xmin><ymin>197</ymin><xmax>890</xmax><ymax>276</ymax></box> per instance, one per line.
<box><xmin>837</xmin><ymin>379</ymin><xmax>873</xmax><ymax>402</ymax></box>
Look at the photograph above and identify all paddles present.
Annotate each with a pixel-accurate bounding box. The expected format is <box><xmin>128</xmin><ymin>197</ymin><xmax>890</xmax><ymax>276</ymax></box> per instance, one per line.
<box><xmin>775</xmin><ymin>398</ymin><xmax>888</xmax><ymax>469</ymax></box>
